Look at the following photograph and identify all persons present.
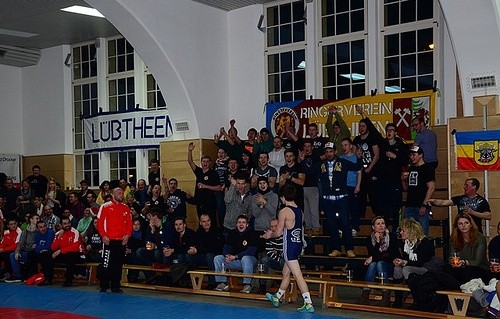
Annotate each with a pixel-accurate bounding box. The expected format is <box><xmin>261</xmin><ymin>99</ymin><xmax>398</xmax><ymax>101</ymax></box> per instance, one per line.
<box><xmin>261</xmin><ymin>184</ymin><xmax>314</xmax><ymax>313</ymax></box>
<box><xmin>96</xmin><ymin>188</ymin><xmax>132</xmax><ymax>294</ymax></box>
<box><xmin>0</xmin><ymin>103</ymin><xmax>500</xmax><ymax>319</ymax></box>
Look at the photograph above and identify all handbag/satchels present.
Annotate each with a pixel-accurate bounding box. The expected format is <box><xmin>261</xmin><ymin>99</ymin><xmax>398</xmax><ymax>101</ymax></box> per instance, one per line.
<box><xmin>482</xmin><ymin>278</ymin><xmax>498</xmax><ymax>292</ymax></box>
<box><xmin>459</xmin><ymin>278</ymin><xmax>485</xmax><ymax>294</ymax></box>
<box><xmin>464</xmin><ymin>288</ymin><xmax>490</xmax><ymax>318</ymax></box>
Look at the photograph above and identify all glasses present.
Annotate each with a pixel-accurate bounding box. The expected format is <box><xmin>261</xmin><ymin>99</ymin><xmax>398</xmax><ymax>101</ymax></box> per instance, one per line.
<box><xmin>412</xmin><ymin>122</ymin><xmax>420</xmax><ymax>127</ymax></box>
<box><xmin>386</xmin><ymin>129</ymin><xmax>395</xmax><ymax>132</ymax></box>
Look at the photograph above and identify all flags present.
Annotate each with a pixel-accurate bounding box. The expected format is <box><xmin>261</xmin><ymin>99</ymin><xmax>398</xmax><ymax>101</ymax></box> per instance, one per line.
<box><xmin>453</xmin><ymin>130</ymin><xmax>500</xmax><ymax>172</ymax></box>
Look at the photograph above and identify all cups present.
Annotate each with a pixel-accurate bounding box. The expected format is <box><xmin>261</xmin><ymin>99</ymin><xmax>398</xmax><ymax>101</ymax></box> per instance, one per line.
<box><xmin>450</xmin><ymin>251</ymin><xmax>460</xmax><ymax>267</ymax></box>
<box><xmin>258</xmin><ymin>264</ymin><xmax>264</xmax><ymax>275</ymax></box>
<box><xmin>347</xmin><ymin>269</ymin><xmax>353</xmax><ymax>282</ymax></box>
<box><xmin>147</xmin><ymin>241</ymin><xmax>152</xmax><ymax>250</ymax></box>
<box><xmin>163</xmin><ymin>245</ymin><xmax>170</xmax><ymax>254</ymax></box>
<box><xmin>221</xmin><ymin>263</ymin><xmax>226</xmax><ymax>272</ymax></box>
<box><xmin>379</xmin><ymin>272</ymin><xmax>386</xmax><ymax>284</ymax></box>
<box><xmin>490</xmin><ymin>258</ymin><xmax>499</xmax><ymax>272</ymax></box>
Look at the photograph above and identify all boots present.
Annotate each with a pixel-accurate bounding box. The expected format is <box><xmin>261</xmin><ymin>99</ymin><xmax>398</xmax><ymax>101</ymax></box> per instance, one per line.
<box><xmin>373</xmin><ymin>291</ymin><xmax>391</xmax><ymax>307</ymax></box>
<box><xmin>392</xmin><ymin>291</ymin><xmax>403</xmax><ymax>308</ymax></box>
<box><xmin>408</xmin><ymin>295</ymin><xmax>423</xmax><ymax>309</ymax></box>
<box><xmin>352</xmin><ymin>290</ymin><xmax>370</xmax><ymax>305</ymax></box>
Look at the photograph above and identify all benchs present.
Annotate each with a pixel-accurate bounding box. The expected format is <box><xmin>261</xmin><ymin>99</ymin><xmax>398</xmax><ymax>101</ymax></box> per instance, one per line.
<box><xmin>80</xmin><ymin>173</ymin><xmax>483</xmax><ymax>319</ymax></box>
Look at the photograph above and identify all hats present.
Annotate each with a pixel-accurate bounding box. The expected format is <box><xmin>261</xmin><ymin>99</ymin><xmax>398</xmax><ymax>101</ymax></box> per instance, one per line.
<box><xmin>409</xmin><ymin>145</ymin><xmax>422</xmax><ymax>155</ymax></box>
<box><xmin>324</xmin><ymin>142</ymin><xmax>336</xmax><ymax>149</ymax></box>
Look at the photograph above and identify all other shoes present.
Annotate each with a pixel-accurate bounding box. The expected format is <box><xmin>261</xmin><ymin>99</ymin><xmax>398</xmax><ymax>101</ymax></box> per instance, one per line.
<box><xmin>297</xmin><ymin>302</ymin><xmax>315</xmax><ymax>313</ymax></box>
<box><xmin>346</xmin><ymin>250</ymin><xmax>356</xmax><ymax>257</ymax></box>
<box><xmin>214</xmin><ymin>283</ymin><xmax>229</xmax><ymax>291</ymax></box>
<box><xmin>351</xmin><ymin>228</ymin><xmax>358</xmax><ymax>237</ymax></box>
<box><xmin>266</xmin><ymin>293</ymin><xmax>279</xmax><ymax>308</ymax></box>
<box><xmin>111</xmin><ymin>288</ymin><xmax>123</xmax><ymax>293</ymax></box>
<box><xmin>328</xmin><ymin>250</ymin><xmax>341</xmax><ymax>257</ymax></box>
<box><xmin>240</xmin><ymin>285</ymin><xmax>252</xmax><ymax>294</ymax></box>
<box><xmin>38</xmin><ymin>278</ymin><xmax>52</xmax><ymax>286</ymax></box>
<box><xmin>5</xmin><ymin>276</ymin><xmax>22</xmax><ymax>283</ymax></box>
